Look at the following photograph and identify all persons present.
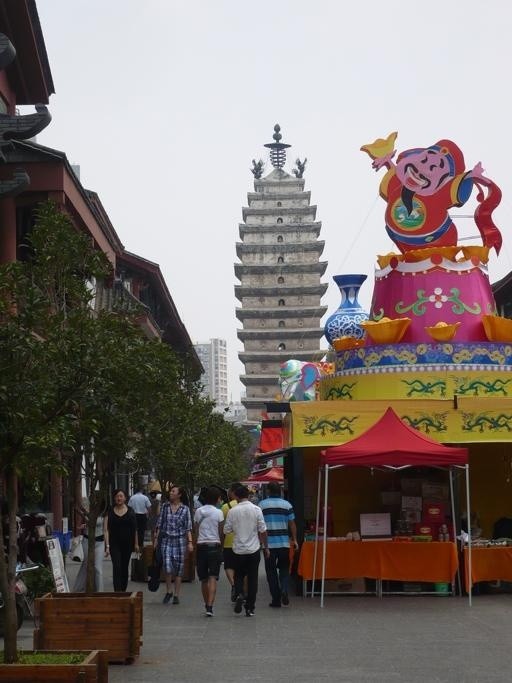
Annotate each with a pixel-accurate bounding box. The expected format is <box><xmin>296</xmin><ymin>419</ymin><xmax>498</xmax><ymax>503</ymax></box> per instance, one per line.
<box><xmin>128</xmin><ymin>482</ymin><xmax>300</xmax><ymax>617</ymax></box>
<box><xmin>102</xmin><ymin>489</ymin><xmax>140</xmax><ymax>594</ymax></box>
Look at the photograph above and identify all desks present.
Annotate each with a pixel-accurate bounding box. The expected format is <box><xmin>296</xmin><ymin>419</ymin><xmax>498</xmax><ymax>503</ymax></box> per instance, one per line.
<box><xmin>463</xmin><ymin>545</ymin><xmax>512</xmax><ymax>596</ymax></box>
<box><xmin>298</xmin><ymin>540</ymin><xmax>461</xmax><ymax>599</ymax></box>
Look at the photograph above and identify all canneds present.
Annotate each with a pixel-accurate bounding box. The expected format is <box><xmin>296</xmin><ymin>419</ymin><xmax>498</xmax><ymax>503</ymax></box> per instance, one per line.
<box><xmin>438</xmin><ymin>524</ymin><xmax>449</xmax><ymax>542</ymax></box>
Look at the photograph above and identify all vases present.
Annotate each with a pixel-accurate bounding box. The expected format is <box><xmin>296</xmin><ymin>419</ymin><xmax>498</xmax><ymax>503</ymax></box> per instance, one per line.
<box><xmin>325</xmin><ymin>273</ymin><xmax>371</xmax><ymax>340</ymax></box>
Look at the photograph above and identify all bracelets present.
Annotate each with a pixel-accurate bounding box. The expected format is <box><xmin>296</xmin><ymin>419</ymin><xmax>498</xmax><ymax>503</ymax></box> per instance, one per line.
<box><xmin>107</xmin><ymin>543</ymin><xmax>109</xmax><ymax>547</ymax></box>
<box><xmin>188</xmin><ymin>539</ymin><xmax>193</xmax><ymax>542</ymax></box>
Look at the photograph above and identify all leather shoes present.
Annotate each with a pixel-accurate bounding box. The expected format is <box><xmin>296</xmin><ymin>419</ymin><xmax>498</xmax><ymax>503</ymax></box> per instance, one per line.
<box><xmin>246</xmin><ymin>608</ymin><xmax>256</xmax><ymax>617</ymax></box>
<box><xmin>234</xmin><ymin>595</ymin><xmax>242</xmax><ymax>613</ymax></box>
<box><xmin>163</xmin><ymin>592</ymin><xmax>172</xmax><ymax>604</ymax></box>
<box><xmin>173</xmin><ymin>596</ymin><xmax>179</xmax><ymax>604</ymax></box>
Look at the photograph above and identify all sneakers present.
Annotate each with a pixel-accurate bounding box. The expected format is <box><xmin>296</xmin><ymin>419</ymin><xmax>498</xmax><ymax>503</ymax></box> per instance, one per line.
<box><xmin>206</xmin><ymin>606</ymin><xmax>215</xmax><ymax>617</ymax></box>
<box><xmin>282</xmin><ymin>590</ymin><xmax>289</xmax><ymax>605</ymax></box>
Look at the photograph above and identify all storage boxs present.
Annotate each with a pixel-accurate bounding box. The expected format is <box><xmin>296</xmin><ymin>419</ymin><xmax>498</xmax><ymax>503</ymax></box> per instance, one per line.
<box><xmin>360</xmin><ymin>465</ymin><xmax>454</xmax><ymax>541</ymax></box>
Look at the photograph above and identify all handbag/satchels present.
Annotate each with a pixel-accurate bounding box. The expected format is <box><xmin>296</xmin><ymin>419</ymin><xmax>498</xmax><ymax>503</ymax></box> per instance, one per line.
<box><xmin>148</xmin><ymin>548</ymin><xmax>160</xmax><ymax>592</ymax></box>
<box><xmin>131</xmin><ymin>551</ymin><xmax>145</xmax><ymax>581</ymax></box>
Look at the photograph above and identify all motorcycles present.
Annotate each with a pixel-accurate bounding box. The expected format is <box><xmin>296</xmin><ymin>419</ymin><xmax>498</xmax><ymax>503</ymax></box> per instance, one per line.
<box><xmin>0</xmin><ymin>573</ymin><xmax>27</xmax><ymax>637</ymax></box>
